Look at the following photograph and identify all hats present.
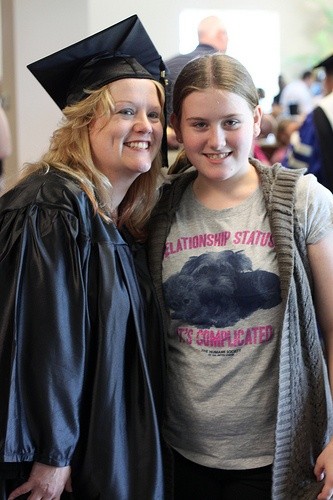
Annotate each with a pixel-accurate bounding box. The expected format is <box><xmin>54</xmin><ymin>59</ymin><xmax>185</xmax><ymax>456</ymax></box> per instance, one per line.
<box><xmin>27</xmin><ymin>14</ymin><xmax>168</xmax><ymax>116</ymax></box>
<box><xmin>314</xmin><ymin>55</ymin><xmax>333</xmax><ymax>75</ymax></box>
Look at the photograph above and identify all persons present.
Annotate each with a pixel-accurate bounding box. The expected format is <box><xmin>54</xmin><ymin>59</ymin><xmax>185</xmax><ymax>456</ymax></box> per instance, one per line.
<box><xmin>0</xmin><ymin>14</ymin><xmax>180</xmax><ymax>500</ymax></box>
<box><xmin>144</xmin><ymin>53</ymin><xmax>333</xmax><ymax>500</ymax></box>
<box><xmin>162</xmin><ymin>19</ymin><xmax>333</xmax><ymax>192</ymax></box>
